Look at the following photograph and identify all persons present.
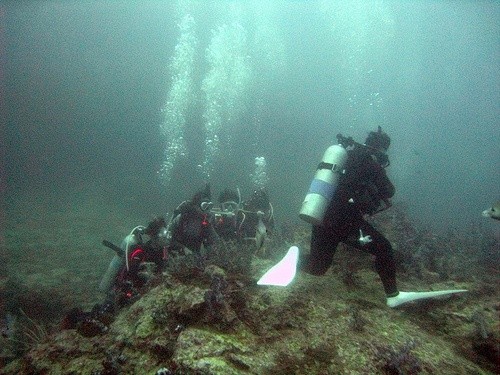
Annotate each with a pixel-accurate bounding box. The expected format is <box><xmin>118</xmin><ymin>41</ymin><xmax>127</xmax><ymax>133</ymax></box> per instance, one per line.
<box><xmin>57</xmin><ymin>214</ymin><xmax>188</xmax><ymax>339</ymax></box>
<box><xmin>155</xmin><ymin>188</ymin><xmax>220</xmax><ymax>275</ymax></box>
<box><xmin>238</xmin><ymin>188</ymin><xmax>276</xmax><ymax>238</ymax></box>
<box><xmin>280</xmin><ymin>124</ymin><xmax>416</xmax><ymax>307</ymax></box>
<box><xmin>203</xmin><ymin>190</ymin><xmax>245</xmax><ymax>252</ymax></box>
